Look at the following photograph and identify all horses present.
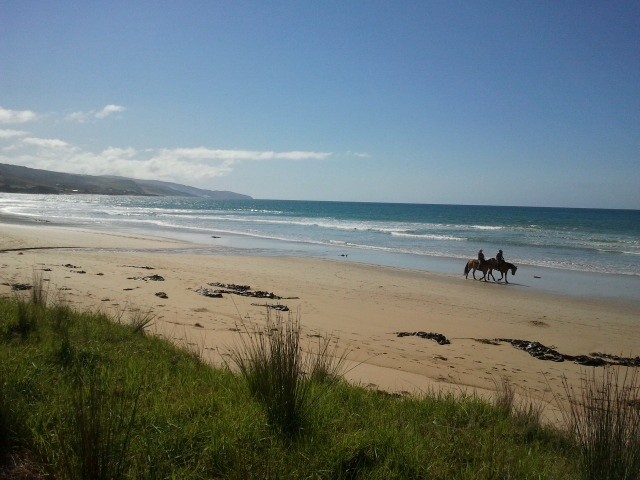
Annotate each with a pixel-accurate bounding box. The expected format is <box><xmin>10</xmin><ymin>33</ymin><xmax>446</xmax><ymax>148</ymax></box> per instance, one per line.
<box><xmin>487</xmin><ymin>259</ymin><xmax>517</xmax><ymax>284</ymax></box>
<box><xmin>464</xmin><ymin>258</ymin><xmax>495</xmax><ymax>282</ymax></box>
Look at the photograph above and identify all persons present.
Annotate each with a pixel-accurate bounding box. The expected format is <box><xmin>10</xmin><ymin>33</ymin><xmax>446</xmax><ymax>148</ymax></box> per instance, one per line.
<box><xmin>477</xmin><ymin>250</ymin><xmax>485</xmax><ymax>269</ymax></box>
<box><xmin>496</xmin><ymin>250</ymin><xmax>504</xmax><ymax>268</ymax></box>
